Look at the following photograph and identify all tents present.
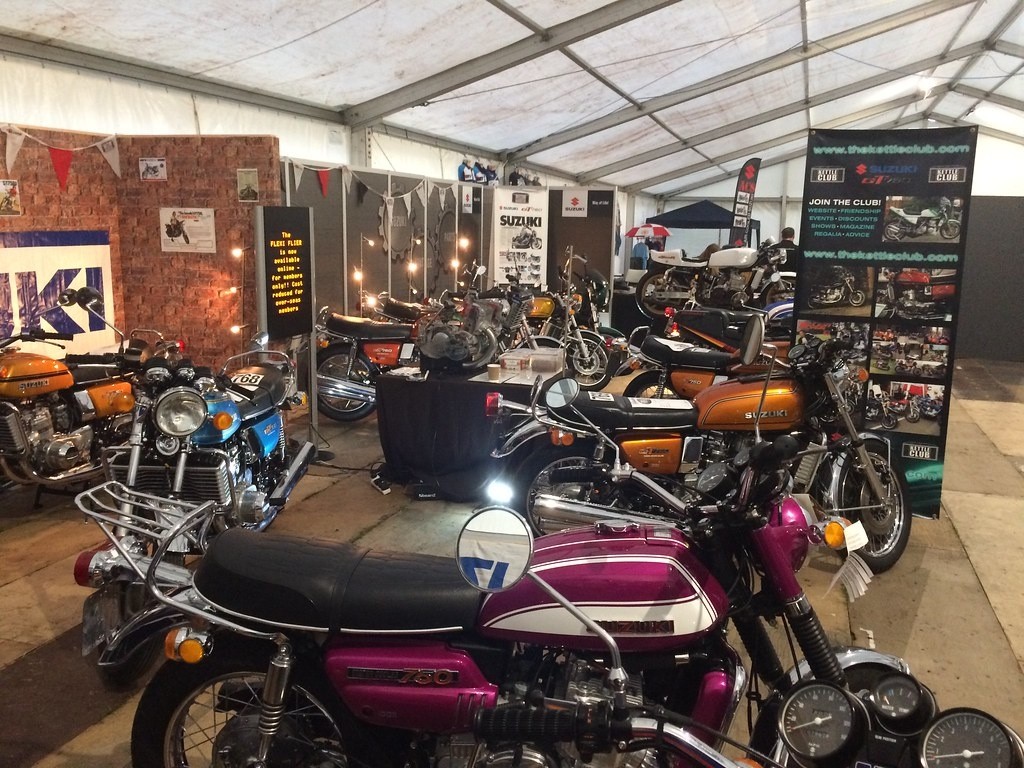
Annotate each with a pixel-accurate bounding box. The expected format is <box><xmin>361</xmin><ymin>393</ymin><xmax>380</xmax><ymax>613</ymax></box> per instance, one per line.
<box><xmin>646</xmin><ymin>199</ymin><xmax>760</xmax><ymax>250</ymax></box>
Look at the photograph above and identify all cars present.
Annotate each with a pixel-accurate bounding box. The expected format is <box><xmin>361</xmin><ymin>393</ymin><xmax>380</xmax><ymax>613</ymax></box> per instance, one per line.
<box><xmin>317</xmin><ymin>306</ymin><xmax>571</xmax><ymax>423</ymax></box>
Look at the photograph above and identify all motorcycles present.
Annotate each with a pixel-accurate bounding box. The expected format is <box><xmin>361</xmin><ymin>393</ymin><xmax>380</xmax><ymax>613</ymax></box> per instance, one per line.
<box><xmin>457</xmin><ymin>506</ymin><xmax>1023</xmax><ymax>767</ymax></box>
<box><xmin>374</xmin><ymin>246</ymin><xmax>629</xmax><ymax>391</ymax></box>
<box><xmin>73</xmin><ymin>349</ymin><xmax>319</xmax><ymax>686</ymax></box>
<box><xmin>624</xmin><ymin>243</ymin><xmax>821</xmax><ymax>404</ymax></box>
<box><xmin>74</xmin><ymin>316</ymin><xmax>924</xmax><ymax>767</ymax></box>
<box><xmin>0</xmin><ymin>328</ymin><xmax>186</xmax><ymax>510</ymax></box>
<box><xmin>488</xmin><ymin>321</ymin><xmax>912</xmax><ymax>574</ymax></box>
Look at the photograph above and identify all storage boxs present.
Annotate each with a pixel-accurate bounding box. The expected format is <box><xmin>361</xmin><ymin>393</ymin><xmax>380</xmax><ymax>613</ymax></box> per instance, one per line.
<box><xmin>529</xmin><ymin>347</ymin><xmax>564</xmax><ymax>373</ymax></box>
<box><xmin>498</xmin><ymin>353</ymin><xmax>528</xmax><ymax>371</ymax></box>
<box><xmin>507</xmin><ymin>348</ymin><xmax>534</xmax><ymax>366</ymax></box>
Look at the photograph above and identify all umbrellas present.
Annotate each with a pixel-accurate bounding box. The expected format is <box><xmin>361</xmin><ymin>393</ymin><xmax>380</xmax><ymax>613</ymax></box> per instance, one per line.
<box><xmin>624</xmin><ymin>222</ymin><xmax>673</xmax><ymax>252</ymax></box>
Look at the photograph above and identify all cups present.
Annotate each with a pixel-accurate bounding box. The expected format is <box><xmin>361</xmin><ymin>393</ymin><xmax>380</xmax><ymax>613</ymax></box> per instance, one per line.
<box><xmin>487</xmin><ymin>364</ymin><xmax>500</xmax><ymax>380</ymax></box>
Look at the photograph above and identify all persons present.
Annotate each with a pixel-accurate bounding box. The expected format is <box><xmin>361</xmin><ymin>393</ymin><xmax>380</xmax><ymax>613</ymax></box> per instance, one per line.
<box><xmin>770</xmin><ymin>226</ymin><xmax>799</xmax><ymax>267</ymax></box>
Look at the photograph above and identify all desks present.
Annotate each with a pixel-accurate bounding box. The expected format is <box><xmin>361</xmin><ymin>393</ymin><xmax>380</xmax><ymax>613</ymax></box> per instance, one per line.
<box><xmin>375</xmin><ymin>361</ymin><xmax>572</xmax><ymax>503</ymax></box>
